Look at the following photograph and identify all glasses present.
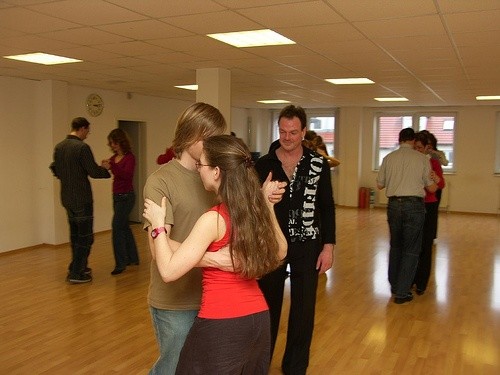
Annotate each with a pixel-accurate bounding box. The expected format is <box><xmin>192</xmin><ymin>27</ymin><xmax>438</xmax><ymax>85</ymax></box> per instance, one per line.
<box><xmin>106</xmin><ymin>141</ymin><xmax>118</xmax><ymax>146</ymax></box>
<box><xmin>195</xmin><ymin>160</ymin><xmax>216</xmax><ymax>169</ymax></box>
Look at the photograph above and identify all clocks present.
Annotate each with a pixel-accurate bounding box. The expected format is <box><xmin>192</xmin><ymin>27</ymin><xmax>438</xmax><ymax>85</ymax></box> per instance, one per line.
<box><xmin>86</xmin><ymin>94</ymin><xmax>104</xmax><ymax>117</ymax></box>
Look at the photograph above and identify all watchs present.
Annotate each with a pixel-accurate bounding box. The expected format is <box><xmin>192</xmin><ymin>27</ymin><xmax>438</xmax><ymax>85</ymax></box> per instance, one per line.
<box><xmin>151</xmin><ymin>226</ymin><xmax>168</xmax><ymax>239</ymax></box>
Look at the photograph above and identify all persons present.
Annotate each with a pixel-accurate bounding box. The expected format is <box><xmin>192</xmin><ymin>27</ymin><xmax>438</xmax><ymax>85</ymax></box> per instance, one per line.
<box><xmin>49</xmin><ymin>116</ymin><xmax>112</xmax><ymax>284</ymax></box>
<box><xmin>426</xmin><ymin>133</ymin><xmax>450</xmax><ymax>239</ymax></box>
<box><xmin>141</xmin><ymin>102</ymin><xmax>288</xmax><ymax>375</ymax></box>
<box><xmin>251</xmin><ymin>103</ymin><xmax>337</xmax><ymax>375</ymax></box>
<box><xmin>143</xmin><ymin>133</ymin><xmax>289</xmax><ymax>375</ymax></box>
<box><xmin>268</xmin><ymin>130</ymin><xmax>341</xmax><ymax>168</ymax></box>
<box><xmin>376</xmin><ymin>126</ymin><xmax>439</xmax><ymax>304</ymax></box>
<box><xmin>409</xmin><ymin>130</ymin><xmax>446</xmax><ymax>295</ymax></box>
<box><xmin>101</xmin><ymin>127</ymin><xmax>140</xmax><ymax>275</ymax></box>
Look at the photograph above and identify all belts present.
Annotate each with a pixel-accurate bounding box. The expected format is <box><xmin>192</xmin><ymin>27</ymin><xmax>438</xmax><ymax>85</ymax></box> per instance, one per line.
<box><xmin>388</xmin><ymin>196</ymin><xmax>424</xmax><ymax>203</ymax></box>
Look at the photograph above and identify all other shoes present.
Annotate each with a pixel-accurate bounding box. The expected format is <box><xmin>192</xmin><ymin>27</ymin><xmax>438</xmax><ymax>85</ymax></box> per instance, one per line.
<box><xmin>126</xmin><ymin>262</ymin><xmax>139</xmax><ymax>266</ymax></box>
<box><xmin>68</xmin><ymin>266</ymin><xmax>91</xmax><ymax>274</ymax></box>
<box><xmin>111</xmin><ymin>265</ymin><xmax>126</xmax><ymax>274</ymax></box>
<box><xmin>394</xmin><ymin>291</ymin><xmax>412</xmax><ymax>303</ymax></box>
<box><xmin>415</xmin><ymin>287</ymin><xmax>424</xmax><ymax>294</ymax></box>
<box><xmin>68</xmin><ymin>271</ymin><xmax>91</xmax><ymax>283</ymax></box>
